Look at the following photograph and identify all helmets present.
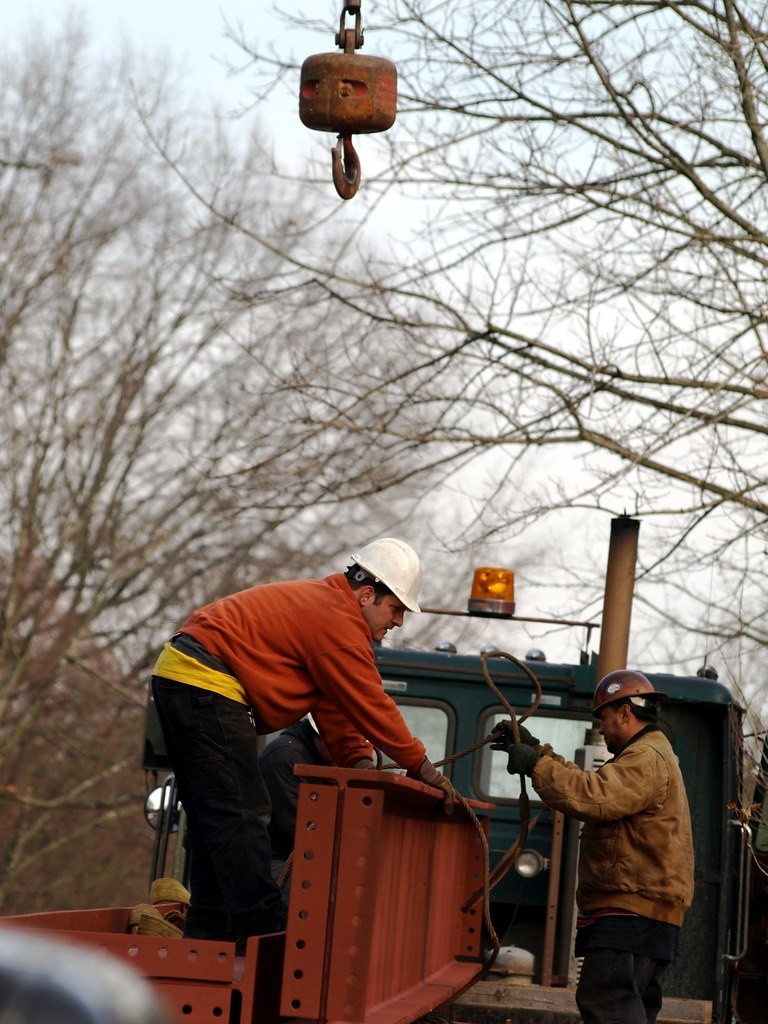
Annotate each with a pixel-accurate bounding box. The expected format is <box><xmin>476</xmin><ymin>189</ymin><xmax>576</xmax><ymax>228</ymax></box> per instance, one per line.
<box><xmin>591</xmin><ymin>670</ymin><xmax>669</xmax><ymax>720</ymax></box>
<box><xmin>352</xmin><ymin>536</ymin><xmax>425</xmax><ymax>615</ymax></box>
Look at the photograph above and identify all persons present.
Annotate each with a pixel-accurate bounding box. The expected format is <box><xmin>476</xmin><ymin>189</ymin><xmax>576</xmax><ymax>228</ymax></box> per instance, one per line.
<box><xmin>485</xmin><ymin>671</ymin><xmax>698</xmax><ymax>1024</ymax></box>
<box><xmin>138</xmin><ymin>535</ymin><xmax>459</xmax><ymax>934</ymax></box>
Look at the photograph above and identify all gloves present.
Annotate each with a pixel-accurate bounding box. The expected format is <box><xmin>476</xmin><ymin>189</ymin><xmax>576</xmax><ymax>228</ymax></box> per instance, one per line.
<box><xmin>489</xmin><ymin>744</ymin><xmax>544</xmax><ymax>777</ymax></box>
<box><xmin>405</xmin><ymin>755</ymin><xmax>455</xmax><ymax>816</ymax></box>
<box><xmin>349</xmin><ymin>759</ymin><xmax>375</xmax><ymax>770</ymax></box>
<box><xmin>487</xmin><ymin>719</ymin><xmax>541</xmax><ymax>750</ymax></box>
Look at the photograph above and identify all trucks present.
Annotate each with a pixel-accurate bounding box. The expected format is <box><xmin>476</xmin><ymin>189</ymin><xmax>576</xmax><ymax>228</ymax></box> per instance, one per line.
<box><xmin>7</xmin><ymin>516</ymin><xmax>760</xmax><ymax>1024</ymax></box>
